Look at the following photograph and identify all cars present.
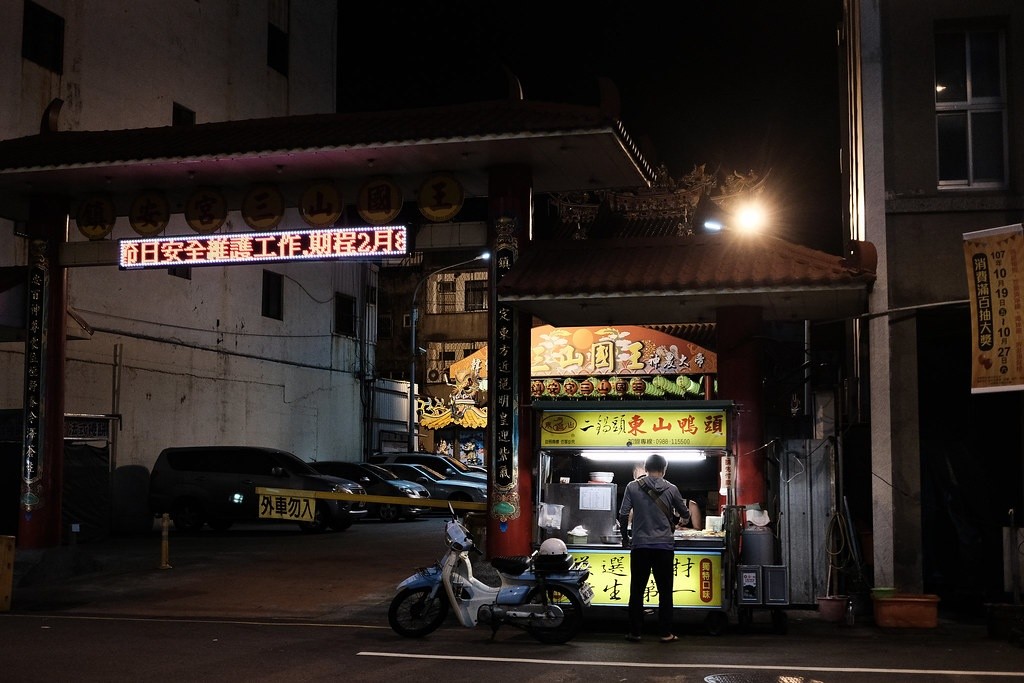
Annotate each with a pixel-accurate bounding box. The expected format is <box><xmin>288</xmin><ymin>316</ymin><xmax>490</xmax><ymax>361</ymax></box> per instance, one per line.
<box><xmin>306</xmin><ymin>451</ymin><xmax>487</xmax><ymax>523</ymax></box>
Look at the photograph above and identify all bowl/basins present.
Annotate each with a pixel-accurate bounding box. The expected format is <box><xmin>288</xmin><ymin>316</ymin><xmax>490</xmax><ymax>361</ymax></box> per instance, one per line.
<box><xmin>873</xmin><ymin>593</ymin><xmax>940</xmax><ymax>628</ymax></box>
<box><xmin>590</xmin><ymin>472</ymin><xmax>614</xmax><ymax>483</ymax></box>
<box><xmin>600</xmin><ymin>535</ymin><xmax>622</xmax><ymax>544</ymax></box>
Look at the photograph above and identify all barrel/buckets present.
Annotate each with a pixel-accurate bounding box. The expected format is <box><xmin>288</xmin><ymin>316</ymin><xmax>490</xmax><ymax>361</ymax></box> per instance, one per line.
<box><xmin>871</xmin><ymin>587</ymin><xmax>896</xmax><ymax>599</ymax></box>
<box><xmin>817</xmin><ymin>596</ymin><xmax>849</xmax><ymax>623</ymax></box>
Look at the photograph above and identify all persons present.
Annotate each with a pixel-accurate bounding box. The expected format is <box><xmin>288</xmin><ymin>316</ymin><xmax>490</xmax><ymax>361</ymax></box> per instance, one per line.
<box><xmin>674</xmin><ymin>497</ymin><xmax>700</xmax><ymax>531</ymax></box>
<box><xmin>618</xmin><ymin>454</ymin><xmax>691</xmax><ymax>643</ymax></box>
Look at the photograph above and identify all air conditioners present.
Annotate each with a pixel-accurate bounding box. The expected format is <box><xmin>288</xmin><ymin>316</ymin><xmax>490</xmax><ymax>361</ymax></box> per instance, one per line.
<box><xmin>426</xmin><ymin>368</ymin><xmax>442</xmax><ymax>383</ymax></box>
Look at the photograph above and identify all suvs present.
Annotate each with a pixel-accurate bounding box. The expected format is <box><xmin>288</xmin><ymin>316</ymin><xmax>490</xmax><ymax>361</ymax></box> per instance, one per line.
<box><xmin>148</xmin><ymin>445</ymin><xmax>368</xmax><ymax>535</ymax></box>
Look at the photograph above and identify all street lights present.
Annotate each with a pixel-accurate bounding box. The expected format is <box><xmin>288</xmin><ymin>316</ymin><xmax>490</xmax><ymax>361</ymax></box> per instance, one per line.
<box><xmin>407</xmin><ymin>253</ymin><xmax>490</xmax><ymax>454</ymax></box>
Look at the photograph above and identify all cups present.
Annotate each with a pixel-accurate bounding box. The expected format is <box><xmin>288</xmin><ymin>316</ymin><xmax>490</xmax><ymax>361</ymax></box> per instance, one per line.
<box><xmin>560</xmin><ymin>477</ymin><xmax>570</xmax><ymax>484</ymax></box>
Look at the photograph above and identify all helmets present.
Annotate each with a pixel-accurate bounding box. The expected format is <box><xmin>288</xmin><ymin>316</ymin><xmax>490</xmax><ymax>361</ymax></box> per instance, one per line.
<box><xmin>539</xmin><ymin>538</ymin><xmax>568</xmax><ymax>556</ymax></box>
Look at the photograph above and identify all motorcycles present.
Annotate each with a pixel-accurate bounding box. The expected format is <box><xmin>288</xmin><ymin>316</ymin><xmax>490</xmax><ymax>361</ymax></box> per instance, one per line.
<box><xmin>389</xmin><ymin>501</ymin><xmax>593</xmax><ymax>646</ymax></box>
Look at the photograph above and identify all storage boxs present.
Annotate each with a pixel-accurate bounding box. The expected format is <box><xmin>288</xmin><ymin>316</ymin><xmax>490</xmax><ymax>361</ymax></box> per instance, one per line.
<box><xmin>870</xmin><ymin>592</ymin><xmax>941</xmax><ymax>630</ymax></box>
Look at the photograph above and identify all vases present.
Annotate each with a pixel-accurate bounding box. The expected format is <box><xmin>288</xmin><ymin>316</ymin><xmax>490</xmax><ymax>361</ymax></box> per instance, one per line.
<box><xmin>815</xmin><ymin>594</ymin><xmax>848</xmax><ymax>623</ymax></box>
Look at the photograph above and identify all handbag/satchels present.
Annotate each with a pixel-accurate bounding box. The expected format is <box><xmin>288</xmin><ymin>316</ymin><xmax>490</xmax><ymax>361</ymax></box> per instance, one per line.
<box><xmin>668</xmin><ymin>512</ymin><xmax>681</xmax><ymax>533</ymax></box>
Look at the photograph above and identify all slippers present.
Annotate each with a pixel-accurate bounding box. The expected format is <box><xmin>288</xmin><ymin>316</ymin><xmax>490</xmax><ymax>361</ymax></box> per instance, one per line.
<box><xmin>660</xmin><ymin>634</ymin><xmax>679</xmax><ymax>642</ymax></box>
<box><xmin>624</xmin><ymin>633</ymin><xmax>641</xmax><ymax>642</ymax></box>
